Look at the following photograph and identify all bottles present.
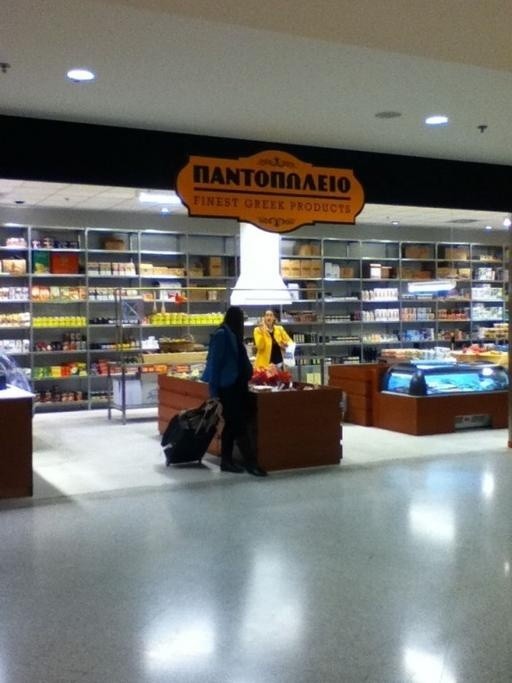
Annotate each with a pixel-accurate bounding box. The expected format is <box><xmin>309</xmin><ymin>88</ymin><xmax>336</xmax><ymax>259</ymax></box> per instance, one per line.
<box><xmin>61</xmin><ymin>333</ymin><xmax>87</xmax><ymax>350</ymax></box>
<box><xmin>34</xmin><ymin>385</ymin><xmax>61</xmax><ymax>402</ymax></box>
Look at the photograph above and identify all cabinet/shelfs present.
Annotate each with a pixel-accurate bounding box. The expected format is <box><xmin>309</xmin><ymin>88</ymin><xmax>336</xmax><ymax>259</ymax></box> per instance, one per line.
<box><xmin>0</xmin><ymin>221</ymin><xmax>509</xmax><ymax>410</ymax></box>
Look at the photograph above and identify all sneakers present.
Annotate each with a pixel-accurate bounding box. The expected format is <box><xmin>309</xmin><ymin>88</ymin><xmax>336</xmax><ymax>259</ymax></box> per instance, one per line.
<box><xmin>221</xmin><ymin>461</ymin><xmax>268</xmax><ymax>477</ymax></box>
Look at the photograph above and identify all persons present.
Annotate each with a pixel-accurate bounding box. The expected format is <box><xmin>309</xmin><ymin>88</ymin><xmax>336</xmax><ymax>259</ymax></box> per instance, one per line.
<box><xmin>254</xmin><ymin>310</ymin><xmax>296</xmax><ymax>375</ymax></box>
<box><xmin>200</xmin><ymin>305</ymin><xmax>268</xmax><ymax>477</ymax></box>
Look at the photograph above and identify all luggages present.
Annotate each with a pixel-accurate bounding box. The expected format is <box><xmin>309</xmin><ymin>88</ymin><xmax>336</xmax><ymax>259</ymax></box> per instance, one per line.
<box><xmin>159</xmin><ymin>398</ymin><xmax>222</xmax><ymax>468</ymax></box>
